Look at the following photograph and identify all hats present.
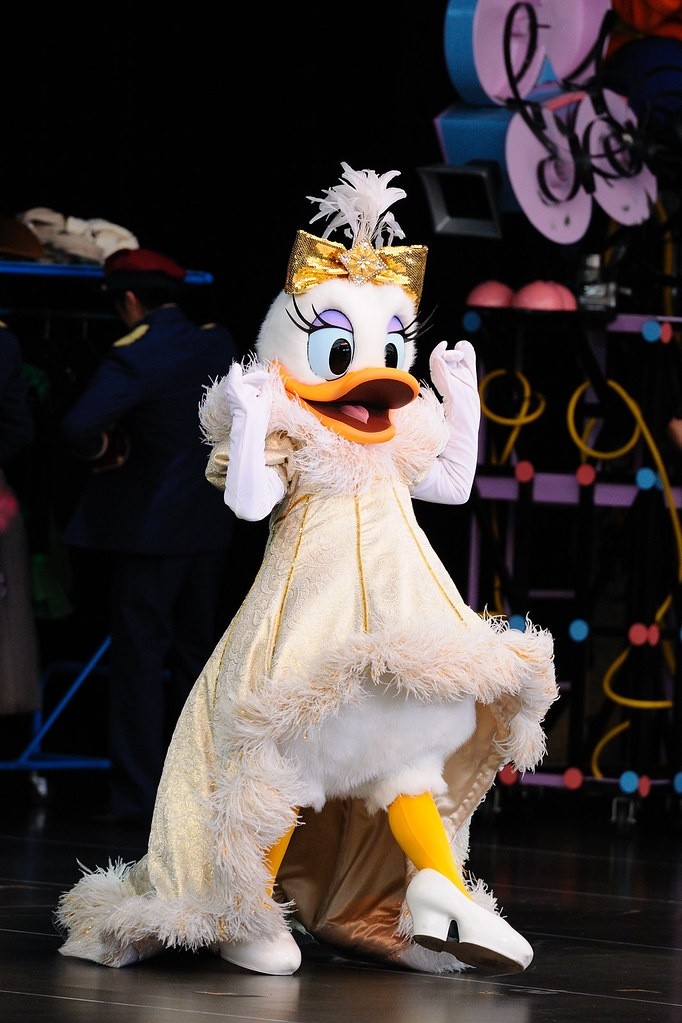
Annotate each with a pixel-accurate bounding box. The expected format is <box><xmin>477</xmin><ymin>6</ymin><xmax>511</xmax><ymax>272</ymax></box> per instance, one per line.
<box><xmin>102</xmin><ymin>250</ymin><xmax>184</xmax><ymax>301</ymax></box>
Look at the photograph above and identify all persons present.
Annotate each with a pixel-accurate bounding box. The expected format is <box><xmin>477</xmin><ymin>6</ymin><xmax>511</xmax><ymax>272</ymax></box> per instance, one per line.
<box><xmin>0</xmin><ymin>321</ymin><xmax>44</xmax><ymax>712</ymax></box>
<box><xmin>71</xmin><ymin>246</ymin><xmax>237</xmax><ymax>823</ymax></box>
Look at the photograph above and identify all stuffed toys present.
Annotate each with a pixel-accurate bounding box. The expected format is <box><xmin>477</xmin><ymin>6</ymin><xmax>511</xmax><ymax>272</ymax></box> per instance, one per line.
<box><xmin>55</xmin><ymin>162</ymin><xmax>558</xmax><ymax>972</ymax></box>
